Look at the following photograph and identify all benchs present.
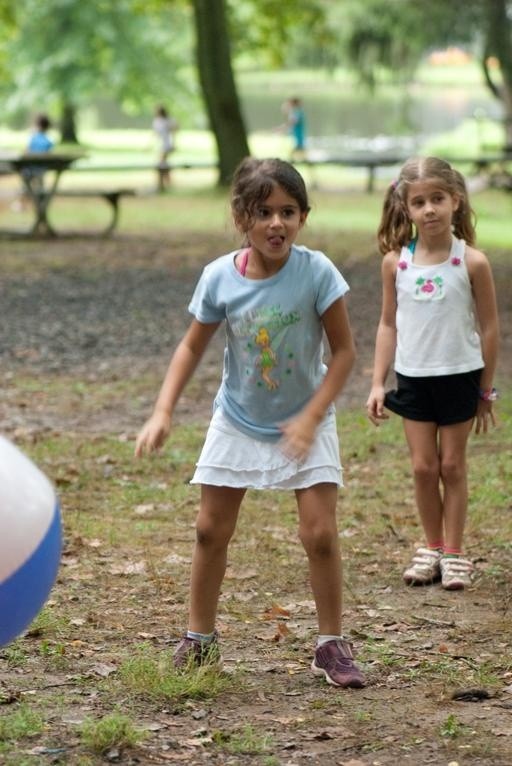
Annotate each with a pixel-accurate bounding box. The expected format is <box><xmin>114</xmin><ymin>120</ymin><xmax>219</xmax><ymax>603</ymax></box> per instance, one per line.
<box><xmin>15</xmin><ymin>184</ymin><xmax>145</xmax><ymax>240</ymax></box>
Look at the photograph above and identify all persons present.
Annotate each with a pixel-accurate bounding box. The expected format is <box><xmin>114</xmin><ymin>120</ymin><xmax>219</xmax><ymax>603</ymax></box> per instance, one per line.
<box><xmin>0</xmin><ymin>429</ymin><xmax>68</xmax><ymax>654</ymax></box>
<box><xmin>277</xmin><ymin>95</ymin><xmax>308</xmax><ymax>165</ymax></box>
<box><xmin>18</xmin><ymin>111</ymin><xmax>51</xmax><ymax>208</ymax></box>
<box><xmin>135</xmin><ymin>155</ymin><xmax>373</xmax><ymax>692</ymax></box>
<box><xmin>364</xmin><ymin>159</ymin><xmax>503</xmax><ymax>590</ymax></box>
<box><xmin>147</xmin><ymin>104</ymin><xmax>177</xmax><ymax>188</ymax></box>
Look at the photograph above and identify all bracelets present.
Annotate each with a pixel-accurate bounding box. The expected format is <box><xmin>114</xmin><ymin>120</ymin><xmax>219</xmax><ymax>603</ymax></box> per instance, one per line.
<box><xmin>479</xmin><ymin>383</ymin><xmax>499</xmax><ymax>402</ymax></box>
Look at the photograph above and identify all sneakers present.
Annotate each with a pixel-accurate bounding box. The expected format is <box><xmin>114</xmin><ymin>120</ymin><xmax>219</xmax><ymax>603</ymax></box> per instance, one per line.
<box><xmin>170</xmin><ymin>630</ymin><xmax>223</xmax><ymax>673</ymax></box>
<box><xmin>309</xmin><ymin>640</ymin><xmax>367</xmax><ymax>690</ymax></box>
<box><xmin>438</xmin><ymin>555</ymin><xmax>474</xmax><ymax>590</ymax></box>
<box><xmin>401</xmin><ymin>546</ymin><xmax>441</xmax><ymax>585</ymax></box>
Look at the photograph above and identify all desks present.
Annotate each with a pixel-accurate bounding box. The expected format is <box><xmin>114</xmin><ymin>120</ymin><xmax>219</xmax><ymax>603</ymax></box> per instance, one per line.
<box><xmin>0</xmin><ymin>154</ymin><xmax>88</xmax><ymax>236</ymax></box>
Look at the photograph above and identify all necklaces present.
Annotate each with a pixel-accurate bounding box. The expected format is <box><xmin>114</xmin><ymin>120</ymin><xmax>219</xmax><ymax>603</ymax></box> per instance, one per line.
<box><xmin>235</xmin><ymin>248</ymin><xmax>259</xmax><ymax>283</ymax></box>
